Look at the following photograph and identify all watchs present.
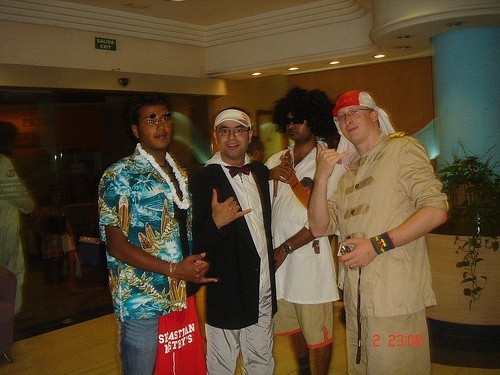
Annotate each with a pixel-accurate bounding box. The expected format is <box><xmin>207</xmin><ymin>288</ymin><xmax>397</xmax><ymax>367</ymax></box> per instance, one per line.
<box><xmin>282</xmin><ymin>240</ymin><xmax>292</xmax><ymax>254</ymax></box>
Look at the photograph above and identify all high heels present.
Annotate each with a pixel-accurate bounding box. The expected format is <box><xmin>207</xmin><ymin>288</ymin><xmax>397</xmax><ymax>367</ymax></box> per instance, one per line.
<box><xmin>1</xmin><ymin>353</ymin><xmax>14</xmax><ymax>363</ymax></box>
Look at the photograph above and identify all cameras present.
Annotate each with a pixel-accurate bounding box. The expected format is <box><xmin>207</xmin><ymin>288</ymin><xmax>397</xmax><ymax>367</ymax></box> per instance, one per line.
<box><xmin>337</xmin><ymin>242</ymin><xmax>355</xmax><ymax>256</ymax></box>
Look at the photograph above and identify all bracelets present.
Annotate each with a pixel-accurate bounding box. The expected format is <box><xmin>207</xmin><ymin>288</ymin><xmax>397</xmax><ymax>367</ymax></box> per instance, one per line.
<box><xmin>370</xmin><ymin>232</ymin><xmax>395</xmax><ymax>255</ymax></box>
<box><xmin>169</xmin><ymin>262</ymin><xmax>177</xmax><ymax>279</ymax></box>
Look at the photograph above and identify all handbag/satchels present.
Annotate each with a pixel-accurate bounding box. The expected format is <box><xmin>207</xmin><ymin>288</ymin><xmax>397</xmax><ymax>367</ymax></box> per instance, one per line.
<box><xmin>61</xmin><ymin>252</ymin><xmax>82</xmax><ymax>277</ymax></box>
<box><xmin>152</xmin><ymin>261</ymin><xmax>208</xmax><ymax>375</ymax></box>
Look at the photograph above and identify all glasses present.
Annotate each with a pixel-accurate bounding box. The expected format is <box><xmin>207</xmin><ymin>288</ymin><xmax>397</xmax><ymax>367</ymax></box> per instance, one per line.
<box><xmin>135</xmin><ymin>112</ymin><xmax>174</xmax><ymax>126</ymax></box>
<box><xmin>283</xmin><ymin>117</ymin><xmax>307</xmax><ymax>125</ymax></box>
<box><xmin>214</xmin><ymin>128</ymin><xmax>248</xmax><ymax>136</ymax></box>
<box><xmin>335</xmin><ymin>108</ymin><xmax>370</xmax><ymax>124</ymax></box>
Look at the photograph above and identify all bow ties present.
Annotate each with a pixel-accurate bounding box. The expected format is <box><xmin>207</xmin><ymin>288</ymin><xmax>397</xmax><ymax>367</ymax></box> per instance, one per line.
<box><xmin>225</xmin><ymin>163</ymin><xmax>252</xmax><ymax>178</ymax></box>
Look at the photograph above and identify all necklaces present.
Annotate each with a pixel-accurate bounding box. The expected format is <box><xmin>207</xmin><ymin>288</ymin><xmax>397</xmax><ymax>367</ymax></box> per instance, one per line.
<box><xmin>137</xmin><ymin>143</ymin><xmax>190</xmax><ymax>209</ymax></box>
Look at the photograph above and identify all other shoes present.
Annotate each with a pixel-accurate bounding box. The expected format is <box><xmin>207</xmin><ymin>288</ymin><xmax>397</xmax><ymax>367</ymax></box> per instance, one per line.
<box><xmin>71</xmin><ymin>288</ymin><xmax>85</xmax><ymax>293</ymax></box>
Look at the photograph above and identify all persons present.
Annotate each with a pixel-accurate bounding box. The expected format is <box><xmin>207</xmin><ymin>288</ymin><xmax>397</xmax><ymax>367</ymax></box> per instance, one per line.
<box><xmin>0</xmin><ymin>120</ymin><xmax>38</xmax><ymax>364</ymax></box>
<box><xmin>98</xmin><ymin>93</ymin><xmax>218</xmax><ymax>375</ymax></box>
<box><xmin>32</xmin><ymin>183</ymin><xmax>81</xmax><ymax>294</ymax></box>
<box><xmin>191</xmin><ymin>107</ymin><xmax>278</xmax><ymax>375</ymax></box>
<box><xmin>264</xmin><ymin>86</ymin><xmax>349</xmax><ymax>375</ymax></box>
<box><xmin>309</xmin><ymin>89</ymin><xmax>449</xmax><ymax>375</ymax></box>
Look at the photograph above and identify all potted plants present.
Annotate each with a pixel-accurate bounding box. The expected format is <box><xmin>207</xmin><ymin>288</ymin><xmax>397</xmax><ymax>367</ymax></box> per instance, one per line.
<box><xmin>424</xmin><ymin>138</ymin><xmax>500</xmax><ymax>310</ymax></box>
